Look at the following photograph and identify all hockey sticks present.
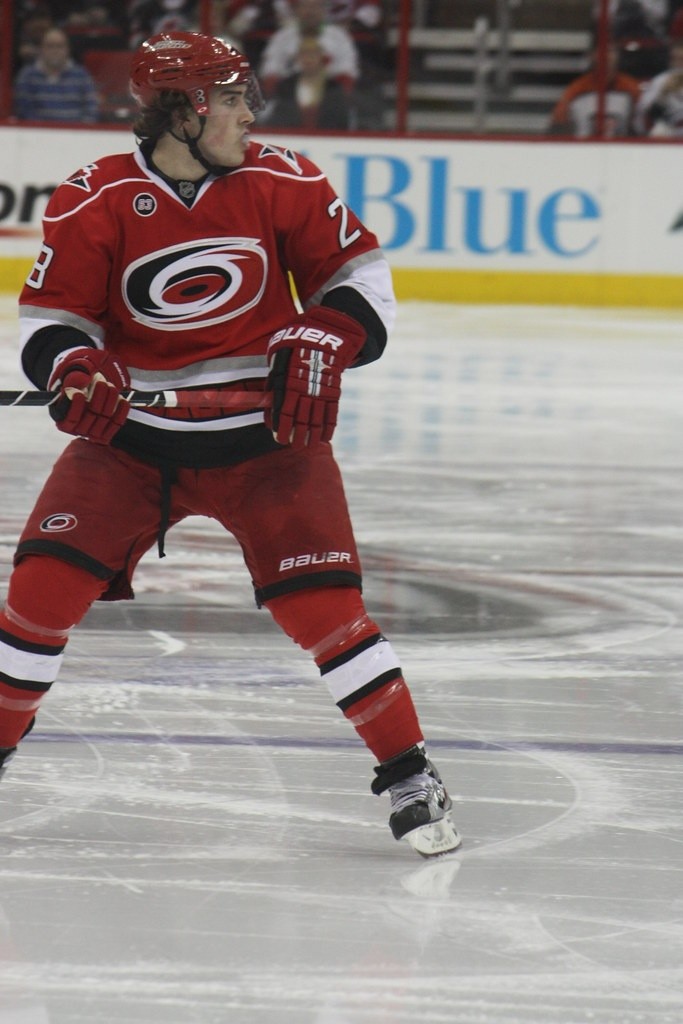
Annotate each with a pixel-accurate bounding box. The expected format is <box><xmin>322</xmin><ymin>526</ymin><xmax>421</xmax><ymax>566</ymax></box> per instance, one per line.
<box><xmin>3</xmin><ymin>385</ymin><xmax>276</xmax><ymax>412</ymax></box>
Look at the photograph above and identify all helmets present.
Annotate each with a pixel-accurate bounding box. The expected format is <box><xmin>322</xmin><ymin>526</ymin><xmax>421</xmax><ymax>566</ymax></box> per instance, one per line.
<box><xmin>130</xmin><ymin>32</ymin><xmax>250</xmax><ymax>108</ymax></box>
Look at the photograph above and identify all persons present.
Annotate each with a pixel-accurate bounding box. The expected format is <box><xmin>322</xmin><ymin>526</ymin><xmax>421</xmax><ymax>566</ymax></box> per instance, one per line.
<box><xmin>547</xmin><ymin>0</ymin><xmax>683</xmax><ymax>138</ymax></box>
<box><xmin>7</xmin><ymin>0</ymin><xmax>401</xmax><ymax>134</ymax></box>
<box><xmin>0</xmin><ymin>30</ymin><xmax>464</xmax><ymax>858</ymax></box>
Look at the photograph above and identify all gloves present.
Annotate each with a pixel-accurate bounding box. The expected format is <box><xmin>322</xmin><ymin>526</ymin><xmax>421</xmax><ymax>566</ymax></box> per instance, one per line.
<box><xmin>46</xmin><ymin>347</ymin><xmax>132</xmax><ymax>444</ymax></box>
<box><xmin>266</xmin><ymin>307</ymin><xmax>365</xmax><ymax>449</ymax></box>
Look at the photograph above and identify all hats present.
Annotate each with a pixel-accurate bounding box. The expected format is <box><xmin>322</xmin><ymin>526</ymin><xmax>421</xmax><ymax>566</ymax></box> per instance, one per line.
<box><xmin>621</xmin><ymin>35</ymin><xmax>662</xmax><ymax>51</ymax></box>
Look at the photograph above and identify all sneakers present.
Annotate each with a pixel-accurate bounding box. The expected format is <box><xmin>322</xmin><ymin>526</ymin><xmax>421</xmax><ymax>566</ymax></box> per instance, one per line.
<box><xmin>372</xmin><ymin>743</ymin><xmax>461</xmax><ymax>857</ymax></box>
